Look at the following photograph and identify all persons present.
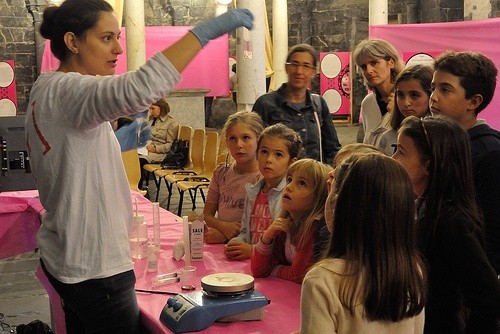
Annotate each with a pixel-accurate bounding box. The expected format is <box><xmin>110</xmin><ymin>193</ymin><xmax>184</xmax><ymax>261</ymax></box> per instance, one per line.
<box><xmin>203</xmin><ymin>110</ymin><xmax>266</xmax><ymax>244</ymax></box>
<box><xmin>23</xmin><ymin>0</ymin><xmax>254</xmax><ymax>334</ymax></box>
<box><xmin>353</xmin><ymin>37</ymin><xmax>406</xmax><ymax>147</ymax></box>
<box><xmin>326</xmin><ymin>143</ymin><xmax>380</xmax><ymax>195</ymax></box>
<box><xmin>251</xmin><ymin>157</ymin><xmax>333</xmax><ymax>285</ymax></box>
<box><xmin>138</xmin><ymin>98</ymin><xmax>179</xmax><ymax>190</ymax></box>
<box><xmin>429</xmin><ymin>50</ymin><xmax>500</xmax><ymax>277</ymax></box>
<box><xmin>223</xmin><ymin>122</ymin><xmax>304</xmax><ymax>261</ymax></box>
<box><xmin>299</xmin><ymin>152</ymin><xmax>428</xmax><ymax>334</ymax></box>
<box><xmin>376</xmin><ymin>64</ymin><xmax>433</xmax><ymax>157</ymax></box>
<box><xmin>391</xmin><ymin>115</ymin><xmax>500</xmax><ymax>334</ymax></box>
<box><xmin>252</xmin><ymin>43</ymin><xmax>343</xmax><ymax>169</ymax></box>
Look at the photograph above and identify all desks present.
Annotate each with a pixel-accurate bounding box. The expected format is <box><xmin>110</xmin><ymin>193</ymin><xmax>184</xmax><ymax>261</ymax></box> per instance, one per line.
<box><xmin>0</xmin><ymin>189</ymin><xmax>302</xmax><ymax>334</ymax></box>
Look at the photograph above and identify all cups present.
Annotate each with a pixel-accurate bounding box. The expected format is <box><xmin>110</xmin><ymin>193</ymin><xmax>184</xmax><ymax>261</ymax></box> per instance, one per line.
<box><xmin>180</xmin><ymin>266</ymin><xmax>196</xmax><ymax>291</ymax></box>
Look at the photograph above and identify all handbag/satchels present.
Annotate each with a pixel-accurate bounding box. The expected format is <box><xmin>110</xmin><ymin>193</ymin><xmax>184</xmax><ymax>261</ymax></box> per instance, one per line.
<box><xmin>160</xmin><ymin>139</ymin><xmax>189</xmax><ymax>169</ymax></box>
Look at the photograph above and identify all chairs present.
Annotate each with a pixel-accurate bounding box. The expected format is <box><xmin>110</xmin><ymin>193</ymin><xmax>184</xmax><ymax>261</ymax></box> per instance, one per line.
<box><xmin>143</xmin><ymin>125</ymin><xmax>235</xmax><ymax>218</ymax></box>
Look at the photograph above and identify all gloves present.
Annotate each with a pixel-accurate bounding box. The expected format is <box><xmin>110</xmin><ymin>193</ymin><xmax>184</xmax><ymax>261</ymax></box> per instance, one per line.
<box><xmin>189</xmin><ymin>9</ymin><xmax>254</xmax><ymax>48</ymax></box>
<box><xmin>115</xmin><ymin>120</ymin><xmax>151</xmax><ymax>151</ymax></box>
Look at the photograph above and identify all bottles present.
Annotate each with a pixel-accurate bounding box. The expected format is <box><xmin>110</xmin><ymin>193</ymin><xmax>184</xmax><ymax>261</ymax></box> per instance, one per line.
<box><xmin>128</xmin><ymin>213</ymin><xmax>148</xmax><ymax>260</ymax></box>
<box><xmin>146</xmin><ymin>245</ymin><xmax>157</xmax><ymax>273</ymax></box>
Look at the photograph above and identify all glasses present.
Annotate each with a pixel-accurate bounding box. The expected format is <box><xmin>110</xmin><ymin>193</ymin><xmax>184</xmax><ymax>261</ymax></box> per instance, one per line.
<box><xmin>285</xmin><ymin>61</ymin><xmax>316</xmax><ymax>73</ymax></box>
